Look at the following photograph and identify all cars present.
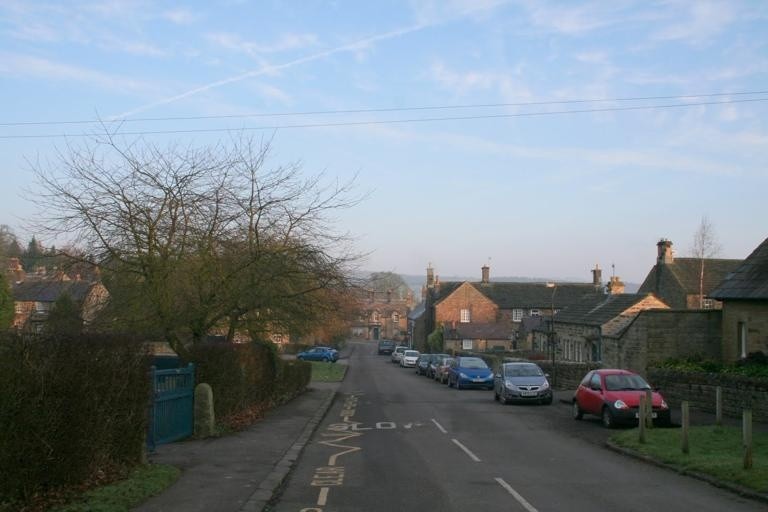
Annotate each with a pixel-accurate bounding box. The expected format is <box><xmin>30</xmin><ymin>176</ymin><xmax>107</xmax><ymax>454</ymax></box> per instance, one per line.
<box><xmin>435</xmin><ymin>358</ymin><xmax>454</xmax><ymax>384</ymax></box>
<box><xmin>415</xmin><ymin>353</ymin><xmax>429</xmax><ymax>375</ymax></box>
<box><xmin>400</xmin><ymin>350</ymin><xmax>420</xmax><ymax>368</ymax></box>
<box><xmin>426</xmin><ymin>355</ymin><xmax>441</xmax><ymax>378</ymax></box>
<box><xmin>493</xmin><ymin>361</ymin><xmax>552</xmax><ymax>406</ymax></box>
<box><xmin>447</xmin><ymin>356</ymin><xmax>494</xmax><ymax>390</ymax></box>
<box><xmin>572</xmin><ymin>369</ymin><xmax>669</xmax><ymax>428</ymax></box>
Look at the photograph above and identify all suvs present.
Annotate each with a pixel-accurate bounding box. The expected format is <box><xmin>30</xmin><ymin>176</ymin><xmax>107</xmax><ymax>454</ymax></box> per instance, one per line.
<box><xmin>379</xmin><ymin>339</ymin><xmax>396</xmax><ymax>355</ymax></box>
<box><xmin>392</xmin><ymin>346</ymin><xmax>410</xmax><ymax>363</ymax></box>
<box><xmin>298</xmin><ymin>346</ymin><xmax>339</xmax><ymax>362</ymax></box>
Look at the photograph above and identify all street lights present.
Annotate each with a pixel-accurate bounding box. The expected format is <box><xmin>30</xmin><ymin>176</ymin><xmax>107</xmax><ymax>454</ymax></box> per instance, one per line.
<box><xmin>546</xmin><ymin>282</ymin><xmax>558</xmax><ymax>386</ymax></box>
